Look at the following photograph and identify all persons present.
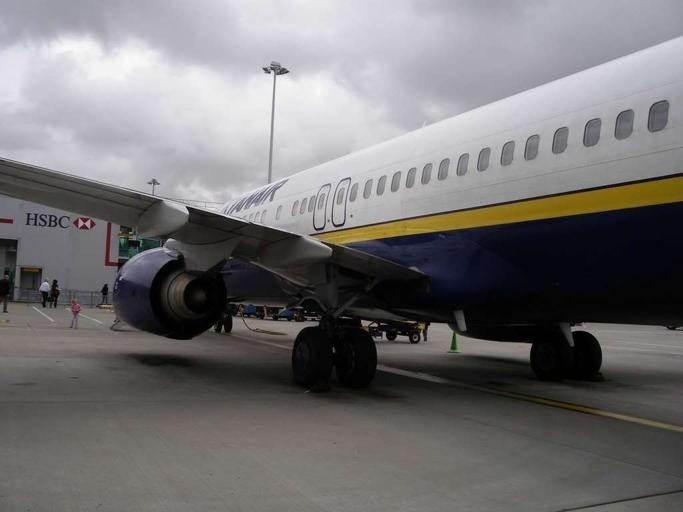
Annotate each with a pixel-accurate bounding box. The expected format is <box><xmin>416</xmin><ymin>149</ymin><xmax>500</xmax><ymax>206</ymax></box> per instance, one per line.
<box><xmin>69</xmin><ymin>299</ymin><xmax>81</xmax><ymax>330</ymax></box>
<box><xmin>49</xmin><ymin>279</ymin><xmax>61</xmax><ymax>308</ymax></box>
<box><xmin>100</xmin><ymin>283</ymin><xmax>110</xmax><ymax>304</ymax></box>
<box><xmin>422</xmin><ymin>322</ymin><xmax>431</xmax><ymax>343</ymax></box>
<box><xmin>0</xmin><ymin>272</ymin><xmax>12</xmax><ymax>314</ymax></box>
<box><xmin>38</xmin><ymin>276</ymin><xmax>52</xmax><ymax>308</ymax></box>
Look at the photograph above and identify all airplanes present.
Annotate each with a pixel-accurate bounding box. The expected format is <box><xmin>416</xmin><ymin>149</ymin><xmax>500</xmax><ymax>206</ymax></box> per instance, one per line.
<box><xmin>0</xmin><ymin>35</ymin><xmax>683</xmax><ymax>397</ymax></box>
